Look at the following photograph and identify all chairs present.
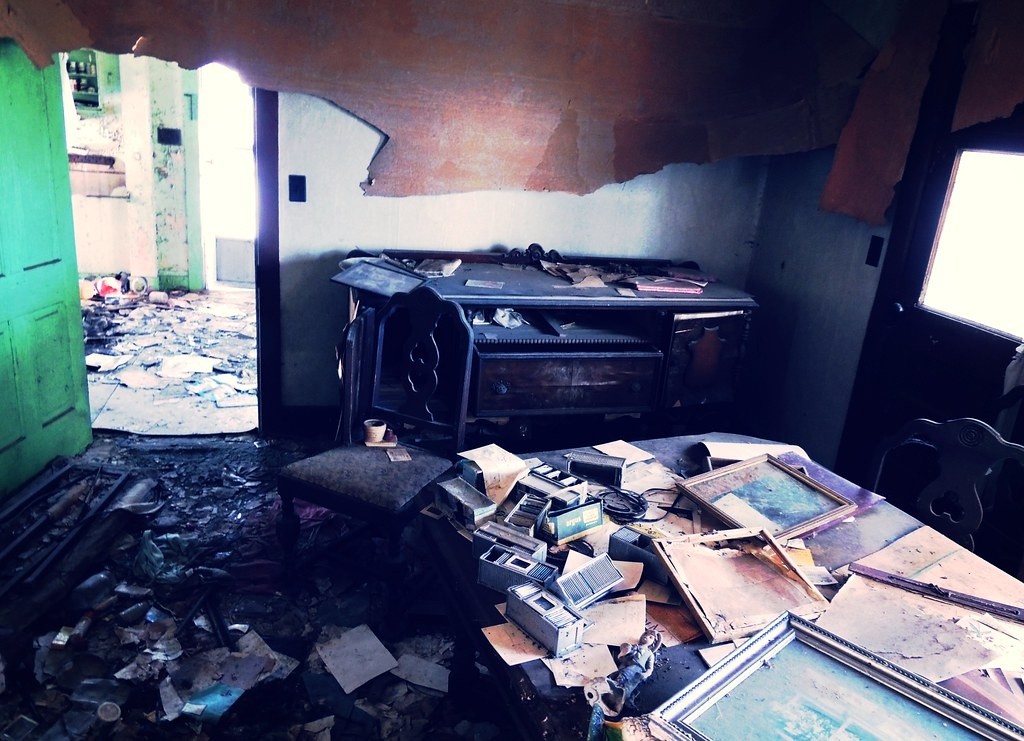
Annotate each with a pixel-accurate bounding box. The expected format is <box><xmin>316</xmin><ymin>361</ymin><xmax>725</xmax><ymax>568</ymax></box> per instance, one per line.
<box><xmin>871</xmin><ymin>418</ymin><xmax>1023</xmax><ymax>585</ymax></box>
<box><xmin>276</xmin><ymin>285</ymin><xmax>475</xmax><ymax>591</ymax></box>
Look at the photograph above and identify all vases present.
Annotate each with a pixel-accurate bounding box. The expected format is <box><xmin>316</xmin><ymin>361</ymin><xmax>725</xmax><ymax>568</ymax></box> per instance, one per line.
<box><xmin>69</xmin><ymin>162</ymin><xmax>126</xmax><ymax>196</ymax></box>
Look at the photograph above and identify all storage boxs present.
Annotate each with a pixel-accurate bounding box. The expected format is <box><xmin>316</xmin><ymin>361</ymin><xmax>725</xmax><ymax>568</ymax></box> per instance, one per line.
<box><xmin>435</xmin><ymin>461</ymin><xmax>625</xmax><ymax>659</ymax></box>
<box><xmin>608</xmin><ymin>526</ymin><xmax>672</xmax><ymax>587</ymax></box>
<box><xmin>567</xmin><ymin>450</ymin><xmax>626</xmax><ymax>490</ymax></box>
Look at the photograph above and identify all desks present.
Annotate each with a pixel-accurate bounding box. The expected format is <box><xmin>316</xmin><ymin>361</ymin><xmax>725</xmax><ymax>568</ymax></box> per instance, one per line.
<box><xmin>421</xmin><ymin>432</ymin><xmax>926</xmax><ymax>741</ymax></box>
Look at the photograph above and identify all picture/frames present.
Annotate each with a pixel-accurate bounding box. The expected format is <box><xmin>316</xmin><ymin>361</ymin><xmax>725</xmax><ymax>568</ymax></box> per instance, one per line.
<box><xmin>649</xmin><ymin>609</ymin><xmax>1023</xmax><ymax>741</ymax></box>
<box><xmin>650</xmin><ymin>528</ymin><xmax>830</xmax><ymax>643</ymax></box>
<box><xmin>674</xmin><ymin>455</ymin><xmax>857</xmax><ymax>545</ymax></box>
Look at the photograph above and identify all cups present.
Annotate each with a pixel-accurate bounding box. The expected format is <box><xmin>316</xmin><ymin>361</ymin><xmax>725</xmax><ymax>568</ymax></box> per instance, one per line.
<box><xmin>364</xmin><ymin>419</ymin><xmax>386</xmax><ymax>442</ymax></box>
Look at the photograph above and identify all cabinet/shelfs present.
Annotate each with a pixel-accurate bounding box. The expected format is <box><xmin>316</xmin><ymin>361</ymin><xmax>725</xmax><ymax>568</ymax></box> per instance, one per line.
<box><xmin>66</xmin><ymin>47</ymin><xmax>123</xmax><ymax>121</ymax></box>
<box><xmin>349</xmin><ymin>250</ymin><xmax>760</xmax><ymax>431</ymax></box>
